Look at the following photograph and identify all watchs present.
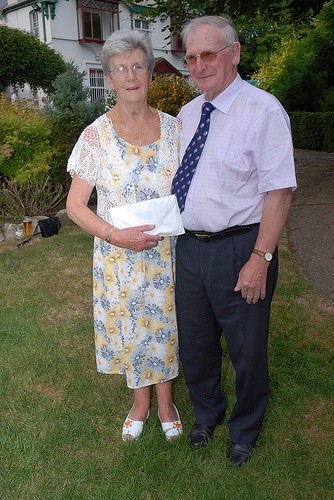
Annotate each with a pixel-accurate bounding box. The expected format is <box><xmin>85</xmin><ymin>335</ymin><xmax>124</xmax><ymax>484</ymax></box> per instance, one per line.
<box><xmin>106</xmin><ymin>227</ymin><xmax>118</xmax><ymax>243</ymax></box>
<box><xmin>251</xmin><ymin>248</ymin><xmax>272</xmax><ymax>262</ymax></box>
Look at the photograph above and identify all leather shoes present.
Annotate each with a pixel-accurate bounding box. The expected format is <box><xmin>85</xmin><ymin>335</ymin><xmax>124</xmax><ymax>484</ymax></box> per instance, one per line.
<box><xmin>190</xmin><ymin>420</ymin><xmax>216</xmax><ymax>448</ymax></box>
<box><xmin>226</xmin><ymin>441</ymin><xmax>253</xmax><ymax>467</ymax></box>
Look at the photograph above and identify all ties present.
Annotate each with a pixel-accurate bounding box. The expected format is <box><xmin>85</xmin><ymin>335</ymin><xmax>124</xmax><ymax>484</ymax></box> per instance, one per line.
<box><xmin>171</xmin><ymin>102</ymin><xmax>217</xmax><ymax>214</ymax></box>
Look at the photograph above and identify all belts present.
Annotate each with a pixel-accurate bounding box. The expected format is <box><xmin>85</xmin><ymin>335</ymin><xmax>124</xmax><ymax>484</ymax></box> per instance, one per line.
<box><xmin>184</xmin><ymin>225</ymin><xmax>254</xmax><ymax>241</ymax></box>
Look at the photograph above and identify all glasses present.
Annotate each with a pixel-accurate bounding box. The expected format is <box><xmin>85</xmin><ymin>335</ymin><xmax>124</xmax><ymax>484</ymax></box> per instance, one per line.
<box><xmin>185</xmin><ymin>42</ymin><xmax>233</xmax><ymax>65</ymax></box>
<box><xmin>108</xmin><ymin>63</ymin><xmax>148</xmax><ymax>78</ymax></box>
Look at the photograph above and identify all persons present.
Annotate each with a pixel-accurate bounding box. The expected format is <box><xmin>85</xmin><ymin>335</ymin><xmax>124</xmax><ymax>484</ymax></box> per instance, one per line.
<box><xmin>64</xmin><ymin>30</ymin><xmax>184</xmax><ymax>445</ymax></box>
<box><xmin>171</xmin><ymin>14</ymin><xmax>298</xmax><ymax>470</ymax></box>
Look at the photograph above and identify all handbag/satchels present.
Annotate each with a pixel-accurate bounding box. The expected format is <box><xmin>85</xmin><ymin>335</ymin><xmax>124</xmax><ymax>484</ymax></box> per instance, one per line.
<box><xmin>109</xmin><ymin>195</ymin><xmax>185</xmax><ymax>236</ymax></box>
<box><xmin>38</xmin><ymin>217</ymin><xmax>58</xmax><ymax>237</ymax></box>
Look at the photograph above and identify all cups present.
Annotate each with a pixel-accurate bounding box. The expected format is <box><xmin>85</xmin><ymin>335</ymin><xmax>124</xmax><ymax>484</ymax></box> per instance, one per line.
<box><xmin>23</xmin><ymin>217</ymin><xmax>33</xmax><ymax>236</ymax></box>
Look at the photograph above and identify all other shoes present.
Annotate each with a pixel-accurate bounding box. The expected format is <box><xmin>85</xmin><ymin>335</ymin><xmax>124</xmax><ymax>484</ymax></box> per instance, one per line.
<box><xmin>122</xmin><ymin>404</ymin><xmax>150</xmax><ymax>442</ymax></box>
<box><xmin>157</xmin><ymin>404</ymin><xmax>183</xmax><ymax>441</ymax></box>
